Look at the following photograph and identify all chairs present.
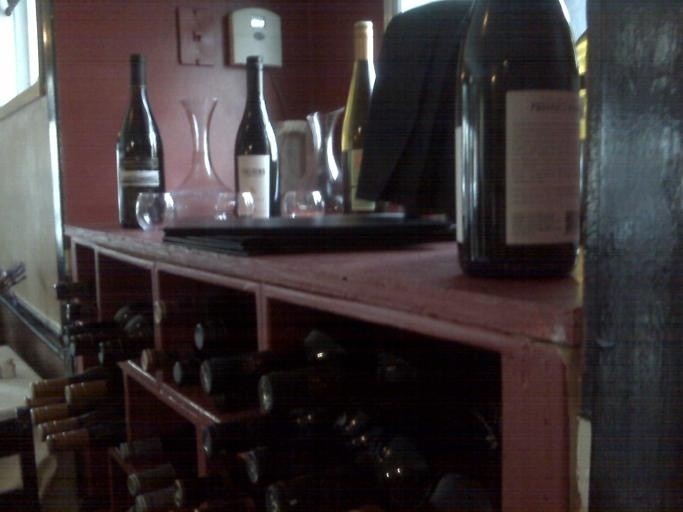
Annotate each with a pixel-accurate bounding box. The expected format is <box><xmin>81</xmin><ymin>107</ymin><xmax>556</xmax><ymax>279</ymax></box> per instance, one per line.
<box><xmin>1</xmin><ymin>406</ymin><xmax>41</xmax><ymax>512</ymax></box>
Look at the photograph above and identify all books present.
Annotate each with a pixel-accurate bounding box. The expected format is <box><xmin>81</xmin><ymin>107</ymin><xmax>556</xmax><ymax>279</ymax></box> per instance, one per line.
<box><xmin>163</xmin><ymin>216</ymin><xmax>450</xmax><ymax>257</ymax></box>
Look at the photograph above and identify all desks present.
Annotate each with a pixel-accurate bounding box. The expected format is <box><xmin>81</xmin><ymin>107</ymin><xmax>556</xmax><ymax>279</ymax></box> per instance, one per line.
<box><xmin>0</xmin><ymin>344</ymin><xmax>84</xmax><ymax>512</ymax></box>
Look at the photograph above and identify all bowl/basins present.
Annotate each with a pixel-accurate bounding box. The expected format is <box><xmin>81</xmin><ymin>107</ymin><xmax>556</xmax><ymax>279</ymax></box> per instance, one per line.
<box><xmin>282</xmin><ymin>189</ymin><xmax>327</xmax><ymax>228</ymax></box>
<box><xmin>134</xmin><ymin>190</ymin><xmax>255</xmax><ymax>232</ymax></box>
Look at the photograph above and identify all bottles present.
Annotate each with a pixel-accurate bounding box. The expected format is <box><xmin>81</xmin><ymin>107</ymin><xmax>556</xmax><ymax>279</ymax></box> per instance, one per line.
<box><xmin>171</xmin><ymin>96</ymin><xmax>233</xmax><ymax>192</ymax></box>
<box><xmin>341</xmin><ymin>20</ymin><xmax>388</xmax><ymax>216</ymax></box>
<box><xmin>27</xmin><ymin>278</ymin><xmax>488</xmax><ymax>511</ymax></box>
<box><xmin>116</xmin><ymin>53</ymin><xmax>166</xmax><ymax>230</ymax></box>
<box><xmin>295</xmin><ymin>105</ymin><xmax>345</xmax><ymax>218</ymax></box>
<box><xmin>452</xmin><ymin>0</ymin><xmax>583</xmax><ymax>283</ymax></box>
<box><xmin>233</xmin><ymin>55</ymin><xmax>281</xmax><ymax>220</ymax></box>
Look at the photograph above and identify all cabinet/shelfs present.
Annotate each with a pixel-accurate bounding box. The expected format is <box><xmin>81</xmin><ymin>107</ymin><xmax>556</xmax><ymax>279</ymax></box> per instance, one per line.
<box><xmin>40</xmin><ymin>0</ymin><xmax>590</xmax><ymax>512</ymax></box>
